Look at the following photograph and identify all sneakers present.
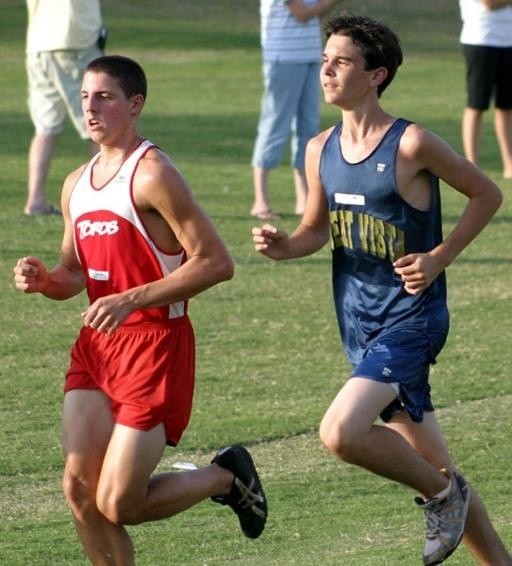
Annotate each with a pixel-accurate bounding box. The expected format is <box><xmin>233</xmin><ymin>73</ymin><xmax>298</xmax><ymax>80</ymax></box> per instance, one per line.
<box><xmin>208</xmin><ymin>446</ymin><xmax>269</xmax><ymax>540</ymax></box>
<box><xmin>414</xmin><ymin>472</ymin><xmax>473</xmax><ymax>566</ymax></box>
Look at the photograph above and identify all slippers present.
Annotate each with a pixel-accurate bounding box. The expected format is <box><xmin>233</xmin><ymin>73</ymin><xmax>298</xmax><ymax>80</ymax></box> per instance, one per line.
<box><xmin>23</xmin><ymin>206</ymin><xmax>62</xmax><ymax>218</ymax></box>
<box><xmin>248</xmin><ymin>207</ymin><xmax>282</xmax><ymax>222</ymax></box>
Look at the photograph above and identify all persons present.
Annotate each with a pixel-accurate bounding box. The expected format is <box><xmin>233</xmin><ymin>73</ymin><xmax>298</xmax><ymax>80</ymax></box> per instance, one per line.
<box><xmin>21</xmin><ymin>0</ymin><xmax>107</xmax><ymax>218</ymax></box>
<box><xmin>246</xmin><ymin>0</ymin><xmax>345</xmax><ymax>222</ymax></box>
<box><xmin>458</xmin><ymin>0</ymin><xmax>512</xmax><ymax>181</ymax></box>
<box><xmin>251</xmin><ymin>8</ymin><xmax>512</xmax><ymax>565</ymax></box>
<box><xmin>14</xmin><ymin>55</ymin><xmax>268</xmax><ymax>564</ymax></box>
